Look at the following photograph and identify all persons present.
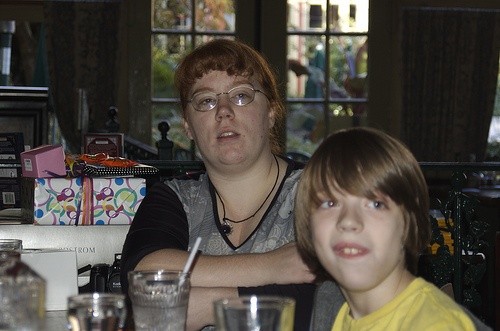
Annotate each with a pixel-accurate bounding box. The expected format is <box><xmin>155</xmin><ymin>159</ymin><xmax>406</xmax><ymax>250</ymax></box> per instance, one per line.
<box><xmin>295</xmin><ymin>128</ymin><xmax>477</xmax><ymax>331</ymax></box>
<box><xmin>120</xmin><ymin>39</ymin><xmax>318</xmax><ymax>331</ymax></box>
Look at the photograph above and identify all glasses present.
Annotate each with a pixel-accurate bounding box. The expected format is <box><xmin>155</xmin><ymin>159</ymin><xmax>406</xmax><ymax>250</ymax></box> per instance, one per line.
<box><xmin>185</xmin><ymin>85</ymin><xmax>271</xmax><ymax>113</ymax></box>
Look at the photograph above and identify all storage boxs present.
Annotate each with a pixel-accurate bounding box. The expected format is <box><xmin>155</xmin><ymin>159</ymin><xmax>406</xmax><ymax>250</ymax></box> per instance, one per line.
<box><xmin>20</xmin><ymin>177</ymin><xmax>146</xmax><ymax>227</ymax></box>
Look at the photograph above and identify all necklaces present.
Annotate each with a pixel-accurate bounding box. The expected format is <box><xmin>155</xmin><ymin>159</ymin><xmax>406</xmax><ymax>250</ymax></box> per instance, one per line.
<box><xmin>215</xmin><ymin>154</ymin><xmax>279</xmax><ymax>235</ymax></box>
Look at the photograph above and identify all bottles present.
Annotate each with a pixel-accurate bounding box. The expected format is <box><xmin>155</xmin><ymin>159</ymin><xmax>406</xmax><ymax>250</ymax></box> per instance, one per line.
<box><xmin>0</xmin><ymin>239</ymin><xmax>47</xmax><ymax>331</ymax></box>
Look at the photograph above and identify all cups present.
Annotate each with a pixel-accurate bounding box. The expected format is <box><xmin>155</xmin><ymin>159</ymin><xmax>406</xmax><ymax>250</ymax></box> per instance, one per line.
<box><xmin>213</xmin><ymin>296</ymin><xmax>296</xmax><ymax>331</ymax></box>
<box><xmin>66</xmin><ymin>294</ymin><xmax>125</xmax><ymax>331</ymax></box>
<box><xmin>128</xmin><ymin>270</ymin><xmax>190</xmax><ymax>331</ymax></box>
